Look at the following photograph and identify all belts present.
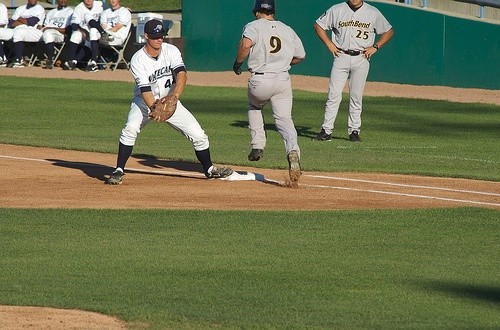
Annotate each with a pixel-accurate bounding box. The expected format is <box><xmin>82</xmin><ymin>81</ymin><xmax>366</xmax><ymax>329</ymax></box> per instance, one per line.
<box><xmin>342</xmin><ymin>50</ymin><xmax>366</xmax><ymax>56</ymax></box>
<box><xmin>251</xmin><ymin>72</ymin><xmax>264</xmax><ymax>75</ymax></box>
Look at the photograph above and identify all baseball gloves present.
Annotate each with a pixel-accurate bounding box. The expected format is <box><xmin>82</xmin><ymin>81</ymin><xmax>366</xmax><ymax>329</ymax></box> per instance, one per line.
<box><xmin>148</xmin><ymin>95</ymin><xmax>177</xmax><ymax>122</ymax></box>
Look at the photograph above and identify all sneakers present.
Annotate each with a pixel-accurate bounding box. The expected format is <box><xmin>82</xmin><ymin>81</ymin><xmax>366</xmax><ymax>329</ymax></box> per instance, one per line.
<box><xmin>107</xmin><ymin>168</ymin><xmax>126</xmax><ymax>184</ymax></box>
<box><xmin>288</xmin><ymin>150</ymin><xmax>302</xmax><ymax>182</ymax></box>
<box><xmin>203</xmin><ymin>165</ymin><xmax>233</xmax><ymax>179</ymax></box>
<box><xmin>318</xmin><ymin>128</ymin><xmax>331</xmax><ymax>141</ymax></box>
<box><xmin>248</xmin><ymin>149</ymin><xmax>263</xmax><ymax>161</ymax></box>
<box><xmin>349</xmin><ymin>130</ymin><xmax>360</xmax><ymax>141</ymax></box>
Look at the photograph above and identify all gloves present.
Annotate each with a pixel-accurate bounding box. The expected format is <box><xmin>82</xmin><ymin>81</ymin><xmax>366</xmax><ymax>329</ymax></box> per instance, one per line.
<box><xmin>233</xmin><ymin>59</ymin><xmax>243</xmax><ymax>75</ymax></box>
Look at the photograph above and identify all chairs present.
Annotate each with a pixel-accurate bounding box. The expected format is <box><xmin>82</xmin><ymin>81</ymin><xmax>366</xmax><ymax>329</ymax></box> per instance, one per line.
<box><xmin>24</xmin><ymin>22</ymin><xmax>135</xmax><ymax>72</ymax></box>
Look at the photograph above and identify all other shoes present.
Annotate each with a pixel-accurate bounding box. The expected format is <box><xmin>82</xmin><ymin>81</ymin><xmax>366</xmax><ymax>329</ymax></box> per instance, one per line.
<box><xmin>0</xmin><ymin>55</ymin><xmax>77</xmax><ymax>70</ymax></box>
<box><xmin>87</xmin><ymin>58</ymin><xmax>99</xmax><ymax>72</ymax></box>
<box><xmin>100</xmin><ymin>33</ymin><xmax>115</xmax><ymax>43</ymax></box>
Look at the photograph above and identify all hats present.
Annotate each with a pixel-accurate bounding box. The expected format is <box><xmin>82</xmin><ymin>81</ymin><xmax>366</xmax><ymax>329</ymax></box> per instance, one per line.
<box><xmin>145</xmin><ymin>19</ymin><xmax>166</xmax><ymax>39</ymax></box>
<box><xmin>253</xmin><ymin>0</ymin><xmax>275</xmax><ymax>13</ymax></box>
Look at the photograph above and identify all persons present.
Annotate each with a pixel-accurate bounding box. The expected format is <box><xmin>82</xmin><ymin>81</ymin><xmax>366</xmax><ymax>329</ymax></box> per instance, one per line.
<box><xmin>0</xmin><ymin>0</ymin><xmax>8</xmax><ymax>64</ymax></box>
<box><xmin>6</xmin><ymin>0</ymin><xmax>74</xmax><ymax>69</ymax></box>
<box><xmin>233</xmin><ymin>0</ymin><xmax>306</xmax><ymax>182</ymax></box>
<box><xmin>313</xmin><ymin>0</ymin><xmax>394</xmax><ymax>142</ymax></box>
<box><xmin>60</xmin><ymin>0</ymin><xmax>103</xmax><ymax>70</ymax></box>
<box><xmin>0</xmin><ymin>0</ymin><xmax>45</xmax><ymax>65</ymax></box>
<box><xmin>65</xmin><ymin>0</ymin><xmax>131</xmax><ymax>72</ymax></box>
<box><xmin>105</xmin><ymin>19</ymin><xmax>233</xmax><ymax>185</ymax></box>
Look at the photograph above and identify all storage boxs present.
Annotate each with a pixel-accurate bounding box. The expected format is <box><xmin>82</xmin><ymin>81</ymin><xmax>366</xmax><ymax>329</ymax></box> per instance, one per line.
<box><xmin>135</xmin><ymin>11</ymin><xmax>164</xmax><ymax>43</ymax></box>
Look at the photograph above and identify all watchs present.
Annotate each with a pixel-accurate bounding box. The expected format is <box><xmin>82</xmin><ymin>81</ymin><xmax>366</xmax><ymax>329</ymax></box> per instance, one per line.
<box><xmin>373</xmin><ymin>44</ymin><xmax>379</xmax><ymax>51</ymax></box>
